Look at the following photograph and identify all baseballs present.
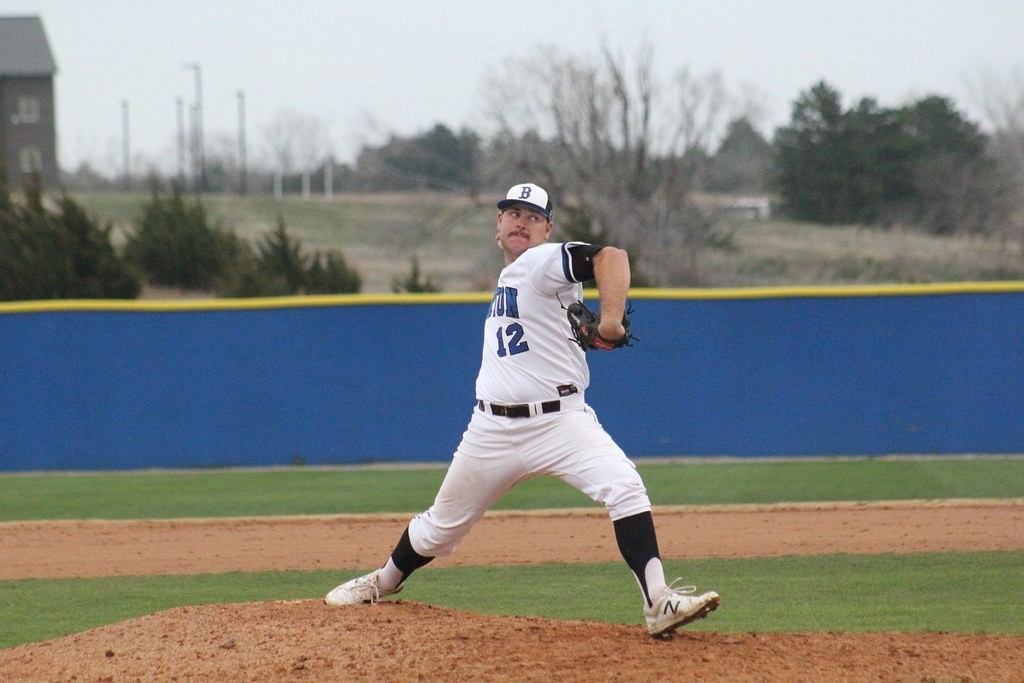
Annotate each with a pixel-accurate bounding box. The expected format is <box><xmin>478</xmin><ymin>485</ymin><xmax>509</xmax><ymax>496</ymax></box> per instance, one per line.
<box><xmin>497</xmin><ymin>239</ymin><xmax>503</xmax><ymax>249</ymax></box>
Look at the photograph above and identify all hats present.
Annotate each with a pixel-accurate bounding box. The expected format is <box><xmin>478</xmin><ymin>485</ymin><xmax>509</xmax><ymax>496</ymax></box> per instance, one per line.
<box><xmin>498</xmin><ymin>183</ymin><xmax>553</xmax><ymax>222</ymax></box>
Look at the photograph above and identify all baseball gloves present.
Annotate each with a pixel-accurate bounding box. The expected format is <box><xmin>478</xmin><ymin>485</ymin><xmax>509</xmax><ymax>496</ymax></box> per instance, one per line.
<box><xmin>566</xmin><ymin>301</ymin><xmax>633</xmax><ymax>351</ymax></box>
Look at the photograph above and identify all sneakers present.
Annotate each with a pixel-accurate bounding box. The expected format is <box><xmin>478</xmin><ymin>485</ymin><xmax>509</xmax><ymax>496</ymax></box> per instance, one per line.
<box><xmin>324</xmin><ymin>564</ymin><xmax>404</xmax><ymax>606</ymax></box>
<box><xmin>644</xmin><ymin>577</ymin><xmax>720</xmax><ymax>639</ymax></box>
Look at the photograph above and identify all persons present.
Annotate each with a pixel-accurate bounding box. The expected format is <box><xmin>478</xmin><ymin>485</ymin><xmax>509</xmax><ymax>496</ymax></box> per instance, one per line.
<box><xmin>323</xmin><ymin>182</ymin><xmax>722</xmax><ymax>638</ymax></box>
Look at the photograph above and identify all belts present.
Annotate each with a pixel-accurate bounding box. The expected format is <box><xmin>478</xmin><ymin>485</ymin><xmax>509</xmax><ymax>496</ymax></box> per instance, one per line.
<box><xmin>479</xmin><ymin>399</ymin><xmax>560</xmax><ymax>418</ymax></box>
